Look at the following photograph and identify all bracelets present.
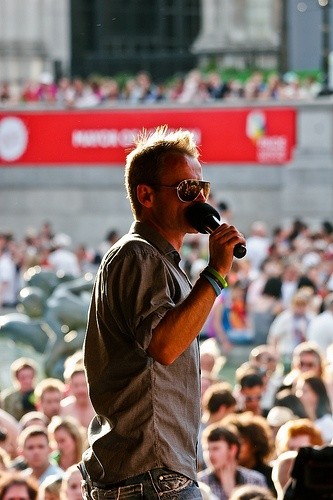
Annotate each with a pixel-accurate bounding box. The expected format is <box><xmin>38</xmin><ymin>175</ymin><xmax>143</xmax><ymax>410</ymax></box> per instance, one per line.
<box><xmin>201</xmin><ymin>266</ymin><xmax>226</xmax><ymax>295</ymax></box>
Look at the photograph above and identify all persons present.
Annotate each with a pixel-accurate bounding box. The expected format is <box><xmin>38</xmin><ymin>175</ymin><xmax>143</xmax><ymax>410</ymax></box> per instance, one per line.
<box><xmin>23</xmin><ymin>428</ymin><xmax>63</xmax><ymax>486</ymax></box>
<box><xmin>50</xmin><ymin>418</ymin><xmax>84</xmax><ymax>472</ymax></box>
<box><xmin>0</xmin><ymin>363</ymin><xmax>37</xmax><ymax>420</ymax></box>
<box><xmin>79</xmin><ymin>133</ymin><xmax>246</xmax><ymax>500</ymax></box>
<box><xmin>61</xmin><ymin>466</ymin><xmax>83</xmax><ymax>500</ymax></box>
<box><xmin>180</xmin><ymin>201</ymin><xmax>333</xmax><ymax>500</ymax></box>
<box><xmin>37</xmin><ymin>378</ymin><xmax>64</xmax><ymax>420</ymax></box>
<box><xmin>0</xmin><ymin>221</ymin><xmax>118</xmax><ymax>379</ymax></box>
<box><xmin>0</xmin><ymin>70</ymin><xmax>333</xmax><ymax>103</ymax></box>
<box><xmin>39</xmin><ymin>476</ymin><xmax>61</xmax><ymax>500</ymax></box>
<box><xmin>20</xmin><ymin>412</ymin><xmax>49</xmax><ymax>431</ymax></box>
<box><xmin>197</xmin><ymin>424</ymin><xmax>267</xmax><ymax>500</ymax></box>
<box><xmin>65</xmin><ymin>369</ymin><xmax>96</xmax><ymax>428</ymax></box>
<box><xmin>0</xmin><ymin>428</ymin><xmax>24</xmax><ymax>474</ymax></box>
<box><xmin>0</xmin><ymin>479</ymin><xmax>36</xmax><ymax>500</ymax></box>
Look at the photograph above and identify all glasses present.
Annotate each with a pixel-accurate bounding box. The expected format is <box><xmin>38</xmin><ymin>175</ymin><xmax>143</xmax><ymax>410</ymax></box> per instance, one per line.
<box><xmin>296</xmin><ymin>361</ymin><xmax>316</xmax><ymax>369</ymax></box>
<box><xmin>243</xmin><ymin>394</ymin><xmax>261</xmax><ymax>403</ymax></box>
<box><xmin>158</xmin><ymin>179</ymin><xmax>211</xmax><ymax>203</ymax></box>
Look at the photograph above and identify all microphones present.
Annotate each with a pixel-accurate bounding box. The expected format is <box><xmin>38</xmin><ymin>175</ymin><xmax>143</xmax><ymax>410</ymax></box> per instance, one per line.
<box><xmin>185</xmin><ymin>202</ymin><xmax>247</xmax><ymax>259</ymax></box>
